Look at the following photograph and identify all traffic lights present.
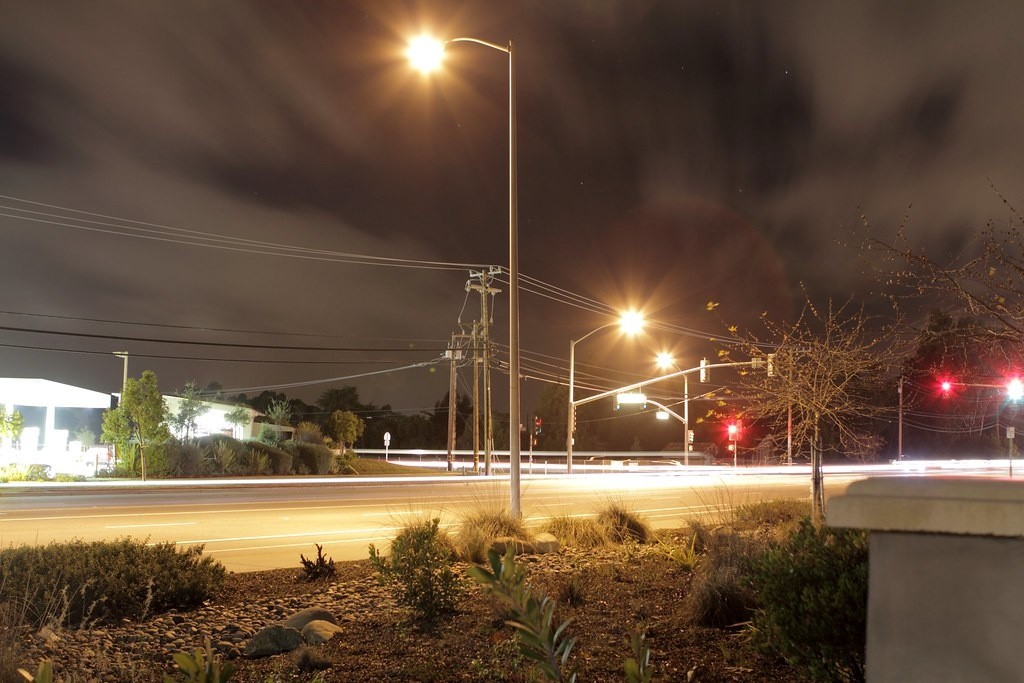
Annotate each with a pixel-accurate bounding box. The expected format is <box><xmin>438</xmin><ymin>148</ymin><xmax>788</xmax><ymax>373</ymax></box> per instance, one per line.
<box><xmin>534</xmin><ymin>417</ymin><xmax>542</xmax><ymax>436</ymax></box>
<box><xmin>729</xmin><ymin>425</ymin><xmax>738</xmax><ymax>441</ymax></box>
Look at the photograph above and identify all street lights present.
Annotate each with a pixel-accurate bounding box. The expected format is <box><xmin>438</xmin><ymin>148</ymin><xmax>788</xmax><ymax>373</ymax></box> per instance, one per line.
<box><xmin>661</xmin><ymin>356</ymin><xmax>688</xmax><ymax>465</ymax></box>
<box><xmin>416</xmin><ymin>38</ymin><xmax>523</xmax><ymax>523</ymax></box>
<box><xmin>567</xmin><ymin>318</ymin><xmax>638</xmax><ymax>474</ymax></box>
